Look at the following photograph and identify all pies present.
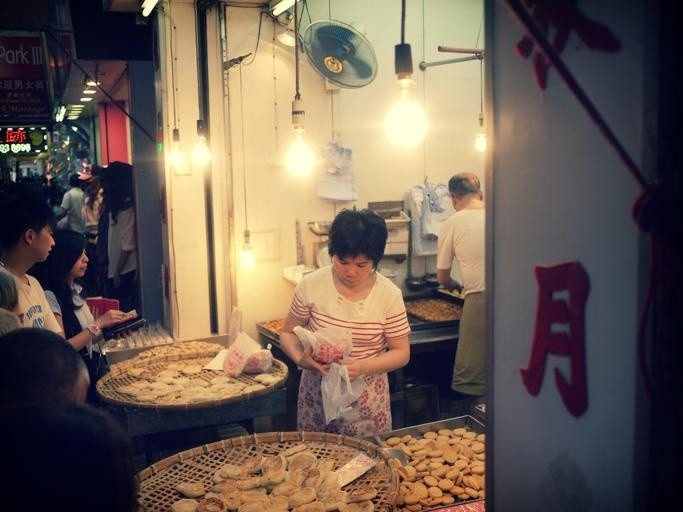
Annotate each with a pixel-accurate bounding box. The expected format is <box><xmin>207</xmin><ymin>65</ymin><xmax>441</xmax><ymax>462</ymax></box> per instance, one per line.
<box><xmin>172</xmin><ymin>444</ymin><xmax>378</xmax><ymax>512</ymax></box>
<box><xmin>117</xmin><ymin>361</ymin><xmax>277</xmax><ymax>404</ymax></box>
<box><xmin>386</xmin><ymin>428</ymin><xmax>486</xmax><ymax>512</ymax></box>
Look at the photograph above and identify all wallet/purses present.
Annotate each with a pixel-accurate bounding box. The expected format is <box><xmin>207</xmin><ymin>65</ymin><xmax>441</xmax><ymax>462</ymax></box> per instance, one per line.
<box><xmin>110</xmin><ymin>315</ymin><xmax>147</xmax><ymax>337</ymax></box>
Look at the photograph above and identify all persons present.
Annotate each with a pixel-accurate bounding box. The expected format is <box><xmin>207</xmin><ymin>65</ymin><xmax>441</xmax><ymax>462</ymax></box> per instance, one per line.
<box><xmin>435</xmin><ymin>174</ymin><xmax>487</xmax><ymax>401</ymax></box>
<box><xmin>279</xmin><ymin>208</ymin><xmax>411</xmax><ymax>438</ymax></box>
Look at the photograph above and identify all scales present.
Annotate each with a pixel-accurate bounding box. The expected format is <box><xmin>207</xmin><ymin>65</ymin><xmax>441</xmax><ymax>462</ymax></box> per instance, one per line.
<box><xmin>307</xmin><ymin>221</ymin><xmax>334</xmax><ymax>268</ymax></box>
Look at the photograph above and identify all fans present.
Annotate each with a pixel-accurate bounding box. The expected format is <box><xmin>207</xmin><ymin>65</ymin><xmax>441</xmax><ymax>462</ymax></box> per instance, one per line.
<box><xmin>301</xmin><ymin>18</ymin><xmax>379</xmax><ymax>90</ymax></box>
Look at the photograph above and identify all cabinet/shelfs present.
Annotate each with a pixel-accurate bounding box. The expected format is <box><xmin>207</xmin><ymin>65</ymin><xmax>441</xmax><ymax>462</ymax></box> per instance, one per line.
<box><xmin>188</xmin><ymin>1</ymin><xmax>211</xmax><ymax>175</ymax></box>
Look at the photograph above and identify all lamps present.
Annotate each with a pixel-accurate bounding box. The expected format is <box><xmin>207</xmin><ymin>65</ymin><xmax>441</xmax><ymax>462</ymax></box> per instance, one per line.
<box><xmin>162</xmin><ymin>0</ymin><xmax>185</xmax><ymax>178</ymax></box>
<box><xmin>276</xmin><ymin>0</ymin><xmax>321</xmax><ymax>184</ymax></box>
<box><xmin>378</xmin><ymin>0</ymin><xmax>433</xmax><ymax>159</ymax></box>
<box><xmin>470</xmin><ymin>12</ymin><xmax>489</xmax><ymax>161</ymax></box>
<box><xmin>267</xmin><ymin>1</ymin><xmax>300</xmax><ymax>19</ymax></box>
<box><xmin>275</xmin><ymin>18</ymin><xmax>300</xmax><ymax>49</ymax></box>
